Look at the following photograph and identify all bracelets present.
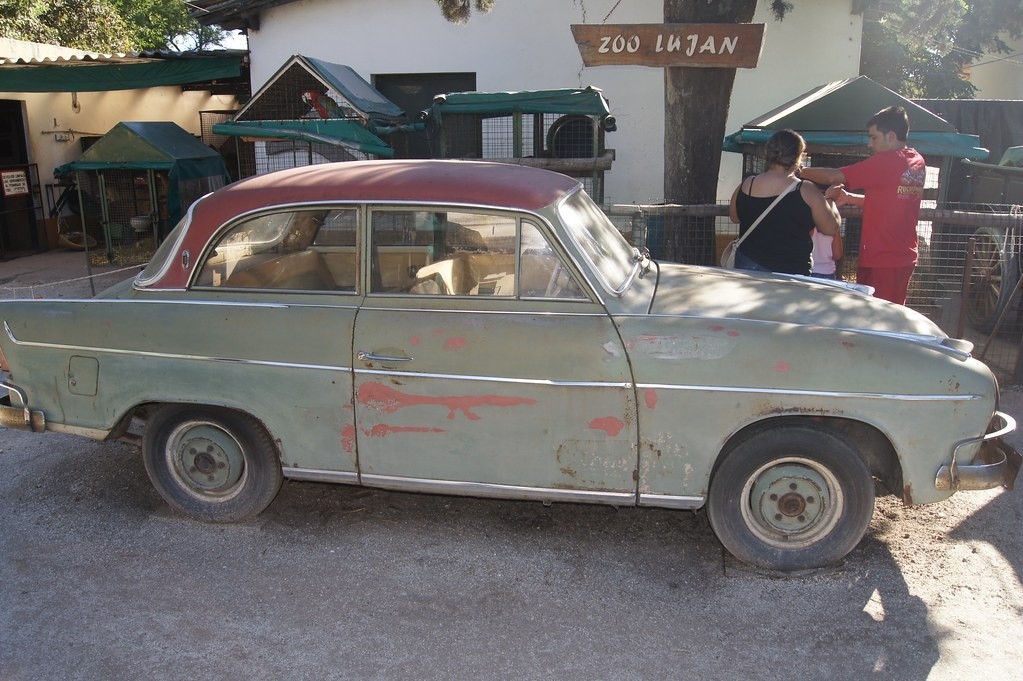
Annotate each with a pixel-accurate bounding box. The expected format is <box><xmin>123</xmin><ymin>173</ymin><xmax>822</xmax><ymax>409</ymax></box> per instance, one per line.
<box><xmin>826</xmin><ymin>198</ymin><xmax>835</xmax><ymax>202</ymax></box>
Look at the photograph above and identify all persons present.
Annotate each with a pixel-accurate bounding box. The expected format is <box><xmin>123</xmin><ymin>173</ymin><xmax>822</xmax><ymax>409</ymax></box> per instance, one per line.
<box><xmin>810</xmin><ymin>228</ymin><xmax>843</xmax><ymax>280</ymax></box>
<box><xmin>729</xmin><ymin>130</ymin><xmax>844</xmax><ymax>277</ymax></box>
<box><xmin>800</xmin><ymin>105</ymin><xmax>926</xmax><ymax>304</ymax></box>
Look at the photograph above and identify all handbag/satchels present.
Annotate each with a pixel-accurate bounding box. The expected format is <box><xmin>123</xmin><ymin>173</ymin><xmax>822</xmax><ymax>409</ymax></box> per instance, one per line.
<box><xmin>720</xmin><ymin>236</ymin><xmax>739</xmax><ymax>270</ymax></box>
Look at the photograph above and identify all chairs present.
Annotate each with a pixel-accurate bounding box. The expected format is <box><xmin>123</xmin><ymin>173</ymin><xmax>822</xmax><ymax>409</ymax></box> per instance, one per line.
<box><xmin>415</xmin><ymin>253</ymin><xmax>480</xmax><ymax>297</ymax></box>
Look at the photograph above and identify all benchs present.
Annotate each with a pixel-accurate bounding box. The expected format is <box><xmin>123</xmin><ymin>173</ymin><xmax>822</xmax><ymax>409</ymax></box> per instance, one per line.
<box><xmin>225</xmin><ymin>248</ymin><xmax>356</xmax><ymax>289</ymax></box>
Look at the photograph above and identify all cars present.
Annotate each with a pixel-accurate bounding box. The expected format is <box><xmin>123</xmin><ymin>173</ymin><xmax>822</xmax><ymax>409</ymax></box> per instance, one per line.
<box><xmin>0</xmin><ymin>157</ymin><xmax>1023</xmax><ymax>573</ymax></box>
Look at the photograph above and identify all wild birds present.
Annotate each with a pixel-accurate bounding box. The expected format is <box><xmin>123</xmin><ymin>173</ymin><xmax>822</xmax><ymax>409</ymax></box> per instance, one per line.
<box><xmin>301</xmin><ymin>90</ymin><xmax>346</xmax><ymax>118</ymax></box>
